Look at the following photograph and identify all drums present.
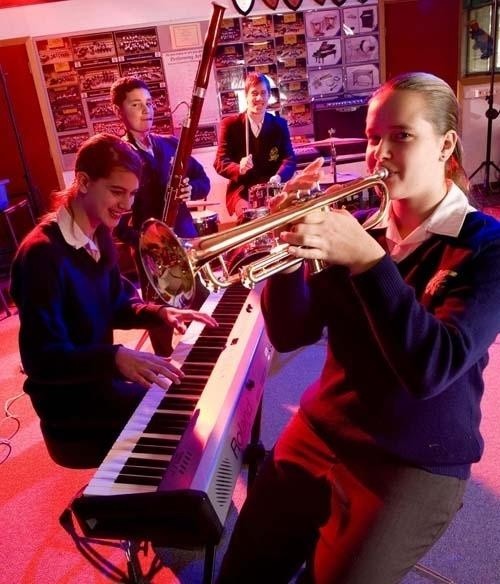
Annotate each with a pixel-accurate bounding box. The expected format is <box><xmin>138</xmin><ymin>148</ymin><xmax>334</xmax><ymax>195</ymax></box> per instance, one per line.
<box><xmin>251</xmin><ymin>183</ymin><xmax>283</xmax><ymax>209</ymax></box>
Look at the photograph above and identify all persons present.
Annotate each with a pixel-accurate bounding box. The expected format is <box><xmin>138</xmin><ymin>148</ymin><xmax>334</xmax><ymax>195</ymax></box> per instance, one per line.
<box><xmin>110</xmin><ymin>77</ymin><xmax>211</xmax><ymax>355</ymax></box>
<box><xmin>214</xmin><ymin>73</ymin><xmax>500</xmax><ymax>584</ymax></box>
<box><xmin>8</xmin><ymin>137</ymin><xmax>219</xmax><ymax>467</ymax></box>
<box><xmin>214</xmin><ymin>71</ymin><xmax>297</xmax><ymax>217</ymax></box>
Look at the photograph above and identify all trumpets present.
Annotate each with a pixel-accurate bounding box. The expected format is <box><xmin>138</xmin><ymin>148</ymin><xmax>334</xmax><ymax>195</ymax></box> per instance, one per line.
<box><xmin>137</xmin><ymin>167</ymin><xmax>390</xmax><ymax>312</ymax></box>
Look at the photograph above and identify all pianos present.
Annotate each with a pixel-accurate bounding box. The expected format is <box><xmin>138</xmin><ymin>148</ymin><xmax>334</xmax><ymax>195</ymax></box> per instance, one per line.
<box><xmin>72</xmin><ymin>281</ymin><xmax>275</xmax><ymax>551</ymax></box>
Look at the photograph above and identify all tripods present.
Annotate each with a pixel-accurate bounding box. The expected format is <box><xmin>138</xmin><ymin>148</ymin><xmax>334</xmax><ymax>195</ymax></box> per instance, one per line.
<box><xmin>469</xmin><ymin>1</ymin><xmax>500</xmax><ymax>198</ymax></box>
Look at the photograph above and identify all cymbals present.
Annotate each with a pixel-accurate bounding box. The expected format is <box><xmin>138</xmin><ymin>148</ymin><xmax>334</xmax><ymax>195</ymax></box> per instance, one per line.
<box><xmin>319</xmin><ymin>171</ymin><xmax>362</xmax><ymax>183</ymax></box>
<box><xmin>295</xmin><ymin>137</ymin><xmax>365</xmax><ymax>149</ymax></box>
